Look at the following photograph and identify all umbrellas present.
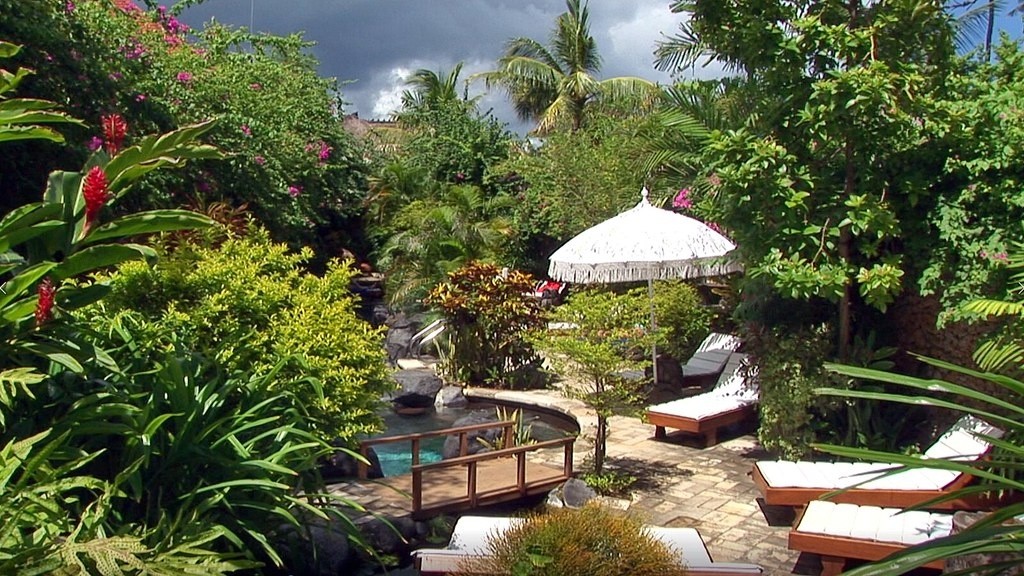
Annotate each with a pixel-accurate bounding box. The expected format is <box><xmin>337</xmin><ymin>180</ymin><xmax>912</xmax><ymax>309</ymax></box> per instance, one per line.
<box><xmin>548</xmin><ymin>199</ymin><xmax>746</xmax><ymax>387</ymax></box>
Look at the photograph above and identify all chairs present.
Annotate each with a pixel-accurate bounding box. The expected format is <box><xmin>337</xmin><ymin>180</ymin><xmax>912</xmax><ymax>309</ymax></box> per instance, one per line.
<box><xmin>642</xmin><ymin>352</ymin><xmax>764</xmax><ymax>448</ymax></box>
<box><xmin>411</xmin><ymin>516</ymin><xmax>764</xmax><ymax>576</ymax></box>
<box><xmin>787</xmin><ymin>500</ymin><xmax>1024</xmax><ymax>576</ymax></box>
<box><xmin>605</xmin><ymin>332</ymin><xmax>747</xmax><ymax>406</ymax></box>
<box><xmin>747</xmin><ymin>412</ymin><xmax>1024</xmax><ymax>527</ymax></box>
